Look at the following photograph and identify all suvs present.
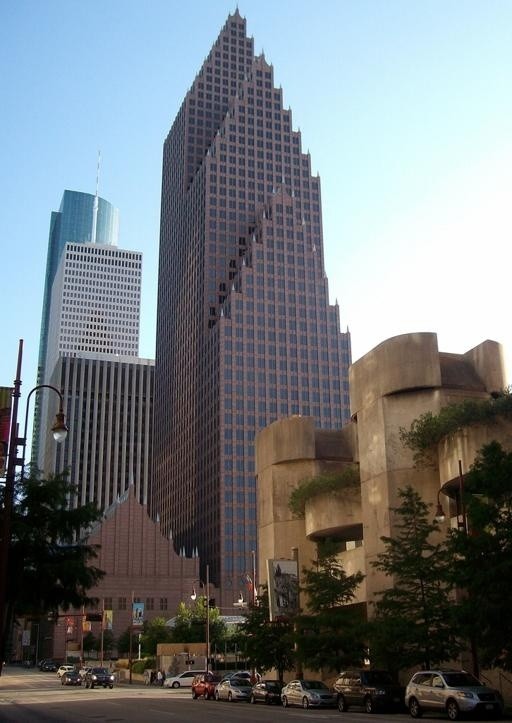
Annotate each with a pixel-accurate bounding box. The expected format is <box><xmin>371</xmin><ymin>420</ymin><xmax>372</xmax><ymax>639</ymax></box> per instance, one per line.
<box><xmin>405</xmin><ymin>667</ymin><xmax>502</xmax><ymax>720</ymax></box>
<box><xmin>331</xmin><ymin>669</ymin><xmax>405</xmax><ymax>713</ymax></box>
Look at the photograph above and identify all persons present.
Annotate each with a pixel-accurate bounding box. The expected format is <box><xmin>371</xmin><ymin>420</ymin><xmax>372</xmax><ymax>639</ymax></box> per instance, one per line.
<box><xmin>143</xmin><ymin>667</ymin><xmax>166</xmax><ymax>689</ymax></box>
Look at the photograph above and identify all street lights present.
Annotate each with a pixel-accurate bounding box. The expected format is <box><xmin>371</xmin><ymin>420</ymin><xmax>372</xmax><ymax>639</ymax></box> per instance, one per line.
<box><xmin>0</xmin><ymin>383</ymin><xmax>70</xmax><ymax>580</ymax></box>
<box><xmin>433</xmin><ymin>484</ymin><xmax>481</xmax><ymax>678</ymax></box>
<box><xmin>190</xmin><ymin>579</ymin><xmax>210</xmax><ymax>666</ymax></box>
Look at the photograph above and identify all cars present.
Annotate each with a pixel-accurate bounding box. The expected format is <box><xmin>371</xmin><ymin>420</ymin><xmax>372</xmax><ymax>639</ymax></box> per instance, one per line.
<box><xmin>214</xmin><ymin>677</ymin><xmax>252</xmax><ymax>701</ymax></box>
<box><xmin>281</xmin><ymin>679</ymin><xmax>336</xmax><ymax>708</ymax></box>
<box><xmin>37</xmin><ymin>658</ymin><xmax>116</xmax><ymax>689</ymax></box>
<box><xmin>191</xmin><ymin>673</ymin><xmax>220</xmax><ymax>700</ymax></box>
<box><xmin>222</xmin><ymin>670</ymin><xmax>263</xmax><ymax>683</ymax></box>
<box><xmin>249</xmin><ymin>679</ymin><xmax>288</xmax><ymax>705</ymax></box>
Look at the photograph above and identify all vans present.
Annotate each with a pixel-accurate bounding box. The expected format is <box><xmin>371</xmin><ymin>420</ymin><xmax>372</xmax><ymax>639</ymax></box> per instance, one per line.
<box><xmin>163</xmin><ymin>669</ymin><xmax>215</xmax><ymax>688</ymax></box>
